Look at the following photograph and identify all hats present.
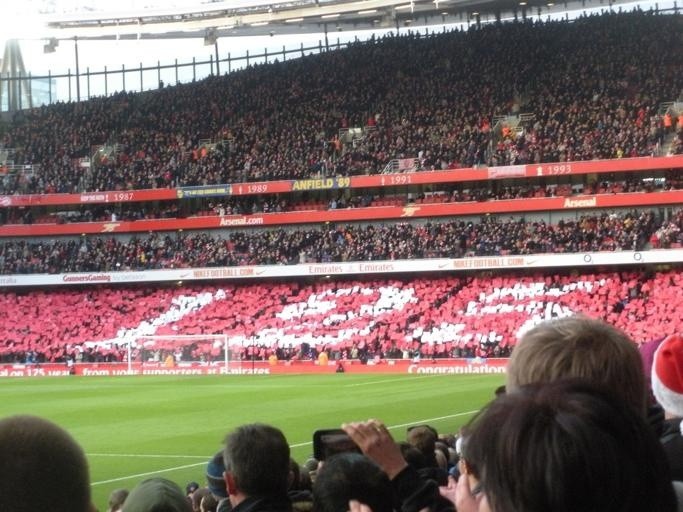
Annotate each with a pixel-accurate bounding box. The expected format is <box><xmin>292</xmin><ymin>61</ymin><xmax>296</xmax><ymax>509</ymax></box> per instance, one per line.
<box><xmin>640</xmin><ymin>334</ymin><xmax>683</xmax><ymax>435</ymax></box>
<box><xmin>109</xmin><ymin>477</ymin><xmax>198</xmax><ymax>512</ymax></box>
<box><xmin>206</xmin><ymin>450</ymin><xmax>227</xmax><ymax>498</ymax></box>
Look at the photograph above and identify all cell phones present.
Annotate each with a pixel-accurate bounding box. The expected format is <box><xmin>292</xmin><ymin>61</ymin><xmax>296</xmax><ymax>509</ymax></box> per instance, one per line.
<box><xmin>313</xmin><ymin>429</ymin><xmax>364</xmax><ymax>461</ymax></box>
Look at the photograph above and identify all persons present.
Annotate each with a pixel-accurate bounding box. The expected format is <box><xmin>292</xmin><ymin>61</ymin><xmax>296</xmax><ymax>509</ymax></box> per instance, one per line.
<box><xmin>1</xmin><ymin>209</ymin><xmax>683</xmax><ymax>274</ymax></box>
<box><xmin>0</xmin><ymin>268</ymin><xmax>682</xmax><ymax>377</ymax></box>
<box><xmin>107</xmin><ymin>314</ymin><xmax>683</xmax><ymax>512</ymax></box>
<box><xmin>1</xmin><ymin>414</ymin><xmax>90</xmax><ymax>511</ymax></box>
<box><xmin>1</xmin><ymin>6</ymin><xmax>682</xmax><ymax>224</ymax></box>
